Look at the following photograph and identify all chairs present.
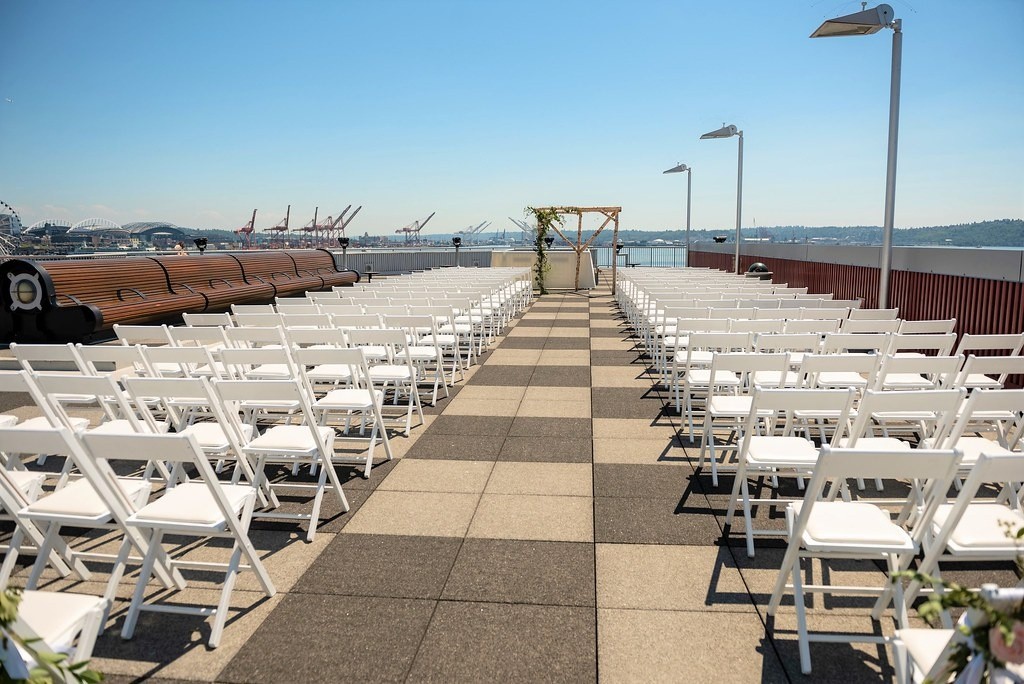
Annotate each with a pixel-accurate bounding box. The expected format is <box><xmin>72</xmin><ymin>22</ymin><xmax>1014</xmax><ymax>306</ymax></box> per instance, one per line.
<box><xmin>616</xmin><ymin>264</ymin><xmax>1024</xmax><ymax>684</ymax></box>
<box><xmin>0</xmin><ymin>265</ymin><xmax>534</xmax><ymax>684</ymax></box>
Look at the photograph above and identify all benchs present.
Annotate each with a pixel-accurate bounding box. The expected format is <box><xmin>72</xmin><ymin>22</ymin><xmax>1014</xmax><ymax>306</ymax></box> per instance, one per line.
<box><xmin>0</xmin><ymin>257</ymin><xmax>206</xmax><ymax>349</ymax></box>
<box><xmin>229</xmin><ymin>253</ymin><xmax>323</xmax><ymax>304</ymax></box>
<box><xmin>284</xmin><ymin>249</ymin><xmax>361</xmax><ymax>292</ymax></box>
<box><xmin>147</xmin><ymin>255</ymin><xmax>275</xmax><ymax>312</ymax></box>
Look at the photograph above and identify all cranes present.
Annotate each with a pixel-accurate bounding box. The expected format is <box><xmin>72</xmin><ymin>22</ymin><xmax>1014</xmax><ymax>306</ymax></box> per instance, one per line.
<box><xmin>395</xmin><ymin>212</ymin><xmax>435</xmax><ymax>246</ymax></box>
<box><xmin>293</xmin><ymin>205</ymin><xmax>362</xmax><ymax>249</ymax></box>
<box><xmin>455</xmin><ymin>220</ymin><xmax>492</xmax><ymax>245</ymax></box>
<box><xmin>508</xmin><ymin>216</ymin><xmax>555</xmax><ymax>244</ymax></box>
<box><xmin>264</xmin><ymin>205</ymin><xmax>291</xmax><ymax>248</ymax></box>
<box><xmin>234</xmin><ymin>208</ymin><xmax>257</xmax><ymax>250</ymax></box>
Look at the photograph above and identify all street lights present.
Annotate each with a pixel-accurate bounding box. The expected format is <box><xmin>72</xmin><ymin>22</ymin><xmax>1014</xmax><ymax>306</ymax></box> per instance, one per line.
<box><xmin>699</xmin><ymin>122</ymin><xmax>744</xmax><ymax>277</ymax></box>
<box><xmin>808</xmin><ymin>2</ymin><xmax>902</xmax><ymax>334</ymax></box>
<box><xmin>663</xmin><ymin>162</ymin><xmax>691</xmax><ymax>246</ymax></box>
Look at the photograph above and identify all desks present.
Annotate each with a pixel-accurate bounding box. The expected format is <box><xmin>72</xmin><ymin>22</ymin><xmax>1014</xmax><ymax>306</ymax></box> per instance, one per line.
<box><xmin>362</xmin><ymin>272</ymin><xmax>379</xmax><ymax>283</ymax></box>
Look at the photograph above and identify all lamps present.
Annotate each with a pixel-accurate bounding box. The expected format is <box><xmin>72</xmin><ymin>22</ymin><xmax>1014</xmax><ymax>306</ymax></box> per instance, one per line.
<box><xmin>713</xmin><ymin>236</ymin><xmax>728</xmax><ymax>244</ymax></box>
<box><xmin>452</xmin><ymin>237</ymin><xmax>461</xmax><ymax>248</ymax></box>
<box><xmin>612</xmin><ymin>244</ymin><xmax>625</xmax><ymax>254</ymax></box>
<box><xmin>544</xmin><ymin>238</ymin><xmax>555</xmax><ymax>249</ymax></box>
<box><xmin>338</xmin><ymin>237</ymin><xmax>349</xmax><ymax>249</ymax></box>
<box><xmin>193</xmin><ymin>237</ymin><xmax>208</xmax><ymax>251</ymax></box>
